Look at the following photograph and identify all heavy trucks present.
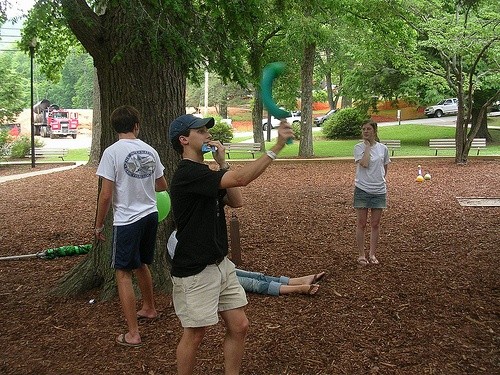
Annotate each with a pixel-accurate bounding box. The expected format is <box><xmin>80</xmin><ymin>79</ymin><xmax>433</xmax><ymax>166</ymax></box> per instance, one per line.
<box><xmin>34</xmin><ymin>109</ymin><xmax>79</xmax><ymax>139</ymax></box>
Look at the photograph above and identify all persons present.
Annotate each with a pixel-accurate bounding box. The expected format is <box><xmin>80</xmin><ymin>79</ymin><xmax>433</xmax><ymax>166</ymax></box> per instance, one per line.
<box><xmin>352</xmin><ymin>120</ymin><xmax>390</xmax><ymax>267</ymax></box>
<box><xmin>168</xmin><ymin>115</ymin><xmax>326</xmax><ymax>375</ymax></box>
<box><xmin>96</xmin><ymin>106</ymin><xmax>169</xmax><ymax>345</ymax></box>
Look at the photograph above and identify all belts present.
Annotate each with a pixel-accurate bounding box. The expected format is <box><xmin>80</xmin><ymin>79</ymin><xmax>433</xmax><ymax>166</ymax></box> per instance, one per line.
<box><xmin>207</xmin><ymin>256</ymin><xmax>224</xmax><ymax>266</ymax></box>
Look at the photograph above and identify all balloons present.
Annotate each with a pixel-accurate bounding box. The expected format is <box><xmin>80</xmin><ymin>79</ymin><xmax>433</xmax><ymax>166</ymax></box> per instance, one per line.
<box><xmin>157</xmin><ymin>189</ymin><xmax>172</xmax><ymax>223</ymax></box>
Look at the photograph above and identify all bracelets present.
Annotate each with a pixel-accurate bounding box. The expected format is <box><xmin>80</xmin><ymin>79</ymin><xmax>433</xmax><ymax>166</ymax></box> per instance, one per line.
<box><xmin>267</xmin><ymin>150</ymin><xmax>279</xmax><ymax>162</ymax></box>
<box><xmin>96</xmin><ymin>224</ymin><xmax>104</xmax><ymax>233</ymax></box>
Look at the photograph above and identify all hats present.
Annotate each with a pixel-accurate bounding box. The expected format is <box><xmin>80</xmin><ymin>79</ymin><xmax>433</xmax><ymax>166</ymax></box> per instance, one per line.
<box><xmin>169</xmin><ymin>114</ymin><xmax>215</xmax><ymax>143</ymax></box>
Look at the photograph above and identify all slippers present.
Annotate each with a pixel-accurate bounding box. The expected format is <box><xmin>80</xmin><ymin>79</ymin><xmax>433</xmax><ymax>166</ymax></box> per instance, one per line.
<box><xmin>358</xmin><ymin>258</ymin><xmax>367</xmax><ymax>264</ymax></box>
<box><xmin>367</xmin><ymin>257</ymin><xmax>379</xmax><ymax>264</ymax></box>
<box><xmin>310</xmin><ymin>272</ymin><xmax>326</xmax><ymax>284</ymax></box>
<box><xmin>116</xmin><ymin>334</ymin><xmax>141</xmax><ymax>347</ymax></box>
<box><xmin>301</xmin><ymin>284</ymin><xmax>320</xmax><ymax>296</ymax></box>
<box><xmin>136</xmin><ymin>312</ymin><xmax>158</xmax><ymax>320</ymax></box>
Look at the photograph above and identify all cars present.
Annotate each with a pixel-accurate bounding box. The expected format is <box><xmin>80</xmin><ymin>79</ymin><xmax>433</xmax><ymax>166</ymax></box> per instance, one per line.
<box><xmin>314</xmin><ymin>109</ymin><xmax>336</xmax><ymax>127</ymax></box>
<box><xmin>424</xmin><ymin>98</ymin><xmax>458</xmax><ymax>118</ymax></box>
<box><xmin>262</xmin><ymin>111</ymin><xmax>300</xmax><ymax>130</ymax></box>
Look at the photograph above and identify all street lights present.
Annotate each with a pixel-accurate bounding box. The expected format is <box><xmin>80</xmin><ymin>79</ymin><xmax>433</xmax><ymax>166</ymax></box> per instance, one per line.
<box><xmin>28</xmin><ymin>37</ymin><xmax>38</xmax><ymax>167</ymax></box>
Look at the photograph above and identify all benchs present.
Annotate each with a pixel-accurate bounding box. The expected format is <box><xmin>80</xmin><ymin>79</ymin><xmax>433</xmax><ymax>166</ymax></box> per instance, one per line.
<box><xmin>429</xmin><ymin>138</ymin><xmax>487</xmax><ymax>156</ymax></box>
<box><xmin>359</xmin><ymin>140</ymin><xmax>400</xmax><ymax>157</ymax></box>
<box><xmin>25</xmin><ymin>148</ymin><xmax>68</xmax><ymax>162</ymax></box>
<box><xmin>223</xmin><ymin>142</ymin><xmax>261</xmax><ymax>159</ymax></box>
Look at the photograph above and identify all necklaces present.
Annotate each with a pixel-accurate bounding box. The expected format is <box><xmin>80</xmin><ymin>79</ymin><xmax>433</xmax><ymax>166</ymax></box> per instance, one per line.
<box><xmin>184</xmin><ymin>157</ymin><xmax>203</xmax><ymax>164</ymax></box>
